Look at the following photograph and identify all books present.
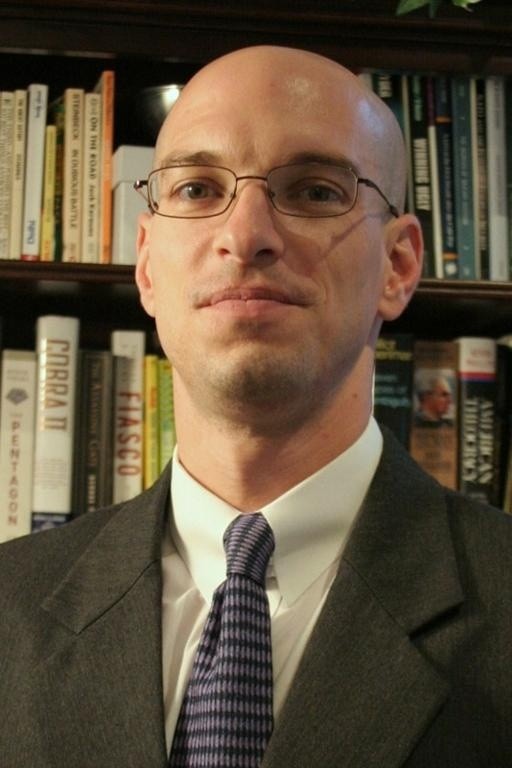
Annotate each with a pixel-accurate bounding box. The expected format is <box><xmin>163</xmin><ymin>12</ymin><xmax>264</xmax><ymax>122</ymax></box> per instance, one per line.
<box><xmin>0</xmin><ymin>315</ymin><xmax>175</xmax><ymax>544</ymax></box>
<box><xmin>360</xmin><ymin>66</ymin><xmax>512</xmax><ymax>280</ymax></box>
<box><xmin>1</xmin><ymin>71</ymin><xmax>114</xmax><ymax>264</ymax></box>
<box><xmin>376</xmin><ymin>336</ymin><xmax>512</xmax><ymax>513</ymax></box>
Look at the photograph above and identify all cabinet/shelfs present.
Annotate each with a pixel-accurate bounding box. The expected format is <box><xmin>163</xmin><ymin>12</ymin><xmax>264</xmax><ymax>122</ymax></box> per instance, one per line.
<box><xmin>1</xmin><ymin>0</ymin><xmax>512</xmax><ymax>297</ymax></box>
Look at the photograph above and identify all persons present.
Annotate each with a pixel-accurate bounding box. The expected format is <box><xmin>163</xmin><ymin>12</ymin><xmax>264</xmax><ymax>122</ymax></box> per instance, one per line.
<box><xmin>0</xmin><ymin>44</ymin><xmax>512</xmax><ymax>768</ymax></box>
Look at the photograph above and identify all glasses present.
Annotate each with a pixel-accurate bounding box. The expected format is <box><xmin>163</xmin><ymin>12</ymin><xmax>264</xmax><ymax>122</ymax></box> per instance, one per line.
<box><xmin>133</xmin><ymin>161</ymin><xmax>401</xmax><ymax>220</ymax></box>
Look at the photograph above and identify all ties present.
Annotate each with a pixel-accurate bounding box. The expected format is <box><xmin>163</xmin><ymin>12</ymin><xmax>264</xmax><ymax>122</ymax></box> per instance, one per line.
<box><xmin>167</xmin><ymin>511</ymin><xmax>275</xmax><ymax>768</ymax></box>
